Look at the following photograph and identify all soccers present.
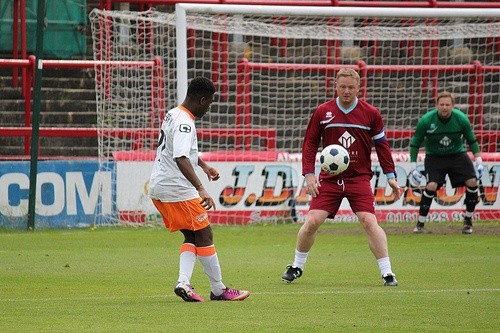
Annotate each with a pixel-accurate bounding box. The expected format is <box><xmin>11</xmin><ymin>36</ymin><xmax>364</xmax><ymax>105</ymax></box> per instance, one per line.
<box><xmin>320</xmin><ymin>145</ymin><xmax>350</xmax><ymax>175</ymax></box>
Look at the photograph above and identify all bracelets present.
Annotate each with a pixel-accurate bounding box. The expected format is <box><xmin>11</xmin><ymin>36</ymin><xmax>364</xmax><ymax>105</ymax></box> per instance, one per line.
<box><xmin>196</xmin><ymin>184</ymin><xmax>202</xmax><ymax>190</ymax></box>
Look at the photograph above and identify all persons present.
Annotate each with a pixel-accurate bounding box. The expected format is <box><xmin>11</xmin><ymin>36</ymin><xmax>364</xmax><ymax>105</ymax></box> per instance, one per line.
<box><xmin>410</xmin><ymin>91</ymin><xmax>483</xmax><ymax>234</ymax></box>
<box><xmin>282</xmin><ymin>68</ymin><xmax>401</xmax><ymax>286</ymax></box>
<box><xmin>149</xmin><ymin>77</ymin><xmax>249</xmax><ymax>303</ymax></box>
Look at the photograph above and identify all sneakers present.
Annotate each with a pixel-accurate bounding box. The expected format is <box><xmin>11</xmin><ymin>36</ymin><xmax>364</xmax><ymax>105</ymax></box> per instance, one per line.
<box><xmin>210</xmin><ymin>287</ymin><xmax>250</xmax><ymax>301</ymax></box>
<box><xmin>174</xmin><ymin>281</ymin><xmax>205</xmax><ymax>302</ymax></box>
<box><xmin>382</xmin><ymin>272</ymin><xmax>398</xmax><ymax>286</ymax></box>
<box><xmin>281</xmin><ymin>265</ymin><xmax>303</xmax><ymax>283</ymax></box>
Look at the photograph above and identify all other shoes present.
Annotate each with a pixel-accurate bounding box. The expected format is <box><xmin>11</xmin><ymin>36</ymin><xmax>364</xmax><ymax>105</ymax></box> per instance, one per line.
<box><xmin>413</xmin><ymin>227</ymin><xmax>424</xmax><ymax>233</ymax></box>
<box><xmin>462</xmin><ymin>226</ymin><xmax>472</xmax><ymax>233</ymax></box>
